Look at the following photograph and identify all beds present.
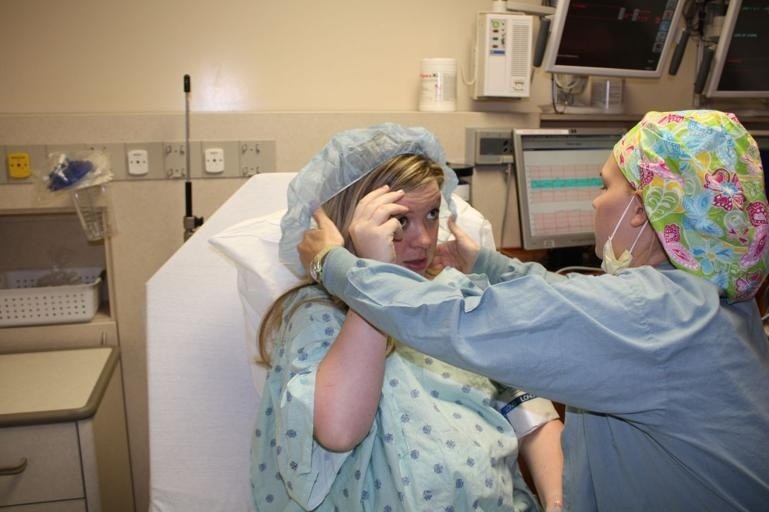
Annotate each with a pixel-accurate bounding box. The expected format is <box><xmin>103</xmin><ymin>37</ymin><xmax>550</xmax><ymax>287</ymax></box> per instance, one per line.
<box><xmin>143</xmin><ymin>172</ymin><xmax>495</xmax><ymax>511</ymax></box>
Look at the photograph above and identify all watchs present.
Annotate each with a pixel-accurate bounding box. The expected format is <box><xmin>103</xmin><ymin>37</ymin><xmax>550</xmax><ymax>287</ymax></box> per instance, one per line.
<box><xmin>307</xmin><ymin>244</ymin><xmax>332</xmax><ymax>289</ymax></box>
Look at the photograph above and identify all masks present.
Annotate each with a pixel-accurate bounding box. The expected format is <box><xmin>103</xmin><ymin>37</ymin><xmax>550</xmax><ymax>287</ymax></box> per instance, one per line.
<box><xmin>601</xmin><ymin>195</ymin><xmax>650</xmax><ymax>276</ymax></box>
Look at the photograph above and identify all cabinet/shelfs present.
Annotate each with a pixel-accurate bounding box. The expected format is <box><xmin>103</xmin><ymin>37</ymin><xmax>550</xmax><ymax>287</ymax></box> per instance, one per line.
<box><xmin>1</xmin><ymin>205</ymin><xmax>134</xmax><ymax>511</ymax></box>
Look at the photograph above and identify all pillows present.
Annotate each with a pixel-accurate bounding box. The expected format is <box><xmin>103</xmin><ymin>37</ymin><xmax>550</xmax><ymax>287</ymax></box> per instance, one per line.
<box><xmin>204</xmin><ymin>193</ymin><xmax>495</xmax><ymax>362</ymax></box>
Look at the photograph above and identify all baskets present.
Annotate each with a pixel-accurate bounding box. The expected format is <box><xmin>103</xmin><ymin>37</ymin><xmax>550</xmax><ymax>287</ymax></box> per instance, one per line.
<box><xmin>0</xmin><ymin>267</ymin><xmax>101</xmax><ymax>327</ymax></box>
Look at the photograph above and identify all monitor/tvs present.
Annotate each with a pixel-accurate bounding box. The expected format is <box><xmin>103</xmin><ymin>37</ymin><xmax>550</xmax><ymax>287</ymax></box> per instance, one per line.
<box><xmin>701</xmin><ymin>0</ymin><xmax>769</xmax><ymax>99</ymax></box>
<box><xmin>542</xmin><ymin>0</ymin><xmax>683</xmax><ymax>79</ymax></box>
<box><xmin>511</xmin><ymin>128</ymin><xmax>633</xmax><ymax>252</ymax></box>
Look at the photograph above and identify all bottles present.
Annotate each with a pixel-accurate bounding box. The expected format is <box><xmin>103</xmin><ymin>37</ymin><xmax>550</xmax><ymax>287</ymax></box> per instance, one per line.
<box><xmin>418</xmin><ymin>57</ymin><xmax>456</xmax><ymax>112</ymax></box>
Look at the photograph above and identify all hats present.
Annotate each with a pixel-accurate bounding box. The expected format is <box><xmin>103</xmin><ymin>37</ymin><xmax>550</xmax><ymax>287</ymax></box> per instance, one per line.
<box><xmin>612</xmin><ymin>109</ymin><xmax>769</xmax><ymax>304</ymax></box>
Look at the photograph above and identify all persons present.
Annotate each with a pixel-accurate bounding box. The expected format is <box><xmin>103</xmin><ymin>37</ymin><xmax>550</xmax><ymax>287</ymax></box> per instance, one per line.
<box><xmin>297</xmin><ymin>108</ymin><xmax>769</xmax><ymax>512</ymax></box>
<box><xmin>249</xmin><ymin>121</ymin><xmax>564</xmax><ymax>512</ymax></box>
